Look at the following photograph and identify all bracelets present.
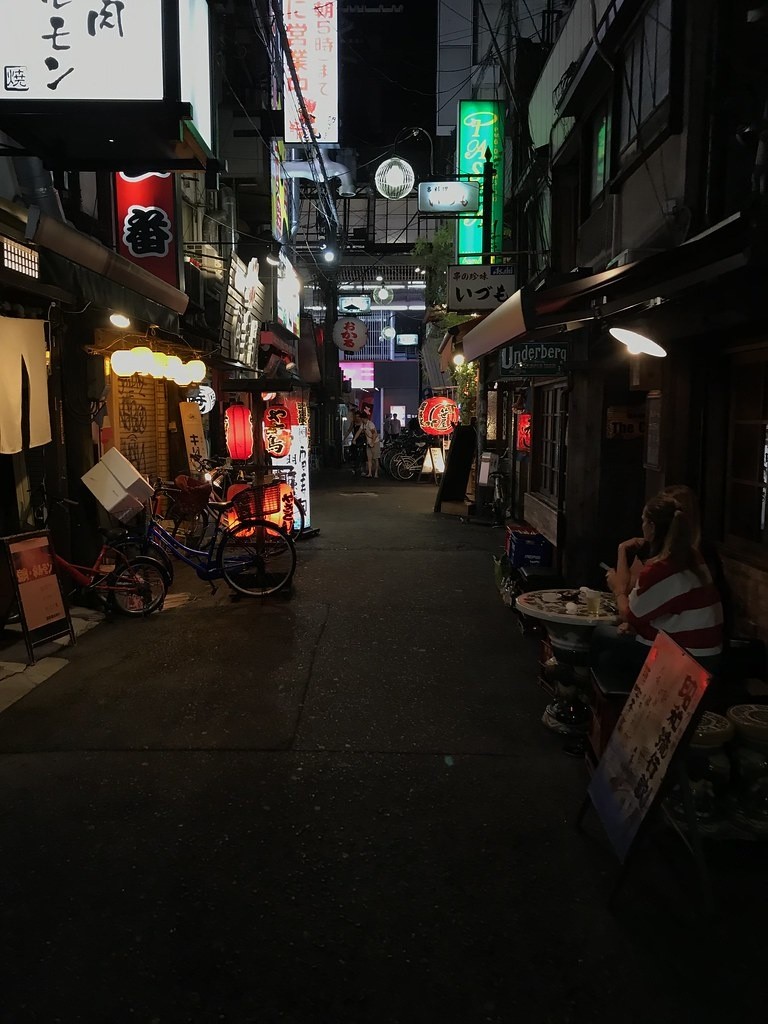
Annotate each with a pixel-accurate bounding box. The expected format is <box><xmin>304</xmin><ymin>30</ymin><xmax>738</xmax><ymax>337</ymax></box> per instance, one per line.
<box><xmin>616</xmin><ymin>592</ymin><xmax>624</xmax><ymax>597</ymax></box>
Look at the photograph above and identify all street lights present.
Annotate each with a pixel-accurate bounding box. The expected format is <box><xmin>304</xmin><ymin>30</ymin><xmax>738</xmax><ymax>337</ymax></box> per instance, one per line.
<box><xmin>331</xmin><ymin>265</ymin><xmax>394</xmax><ymax>469</ymax></box>
<box><xmin>373</xmin><ymin>125</ymin><xmax>497</xmax><ymax>518</ymax></box>
<box><xmin>381</xmin><ymin>314</ymin><xmax>424</xmax><ymax>404</ymax></box>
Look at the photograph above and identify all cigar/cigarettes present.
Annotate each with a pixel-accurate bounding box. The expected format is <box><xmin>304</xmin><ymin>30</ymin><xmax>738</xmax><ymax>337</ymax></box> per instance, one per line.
<box><xmin>600</xmin><ymin>562</ymin><xmax>610</xmax><ymax>570</ymax></box>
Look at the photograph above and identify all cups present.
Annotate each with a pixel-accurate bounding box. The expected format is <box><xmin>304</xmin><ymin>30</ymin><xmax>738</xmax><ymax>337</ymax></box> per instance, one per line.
<box><xmin>580</xmin><ymin>587</ymin><xmax>589</xmax><ymax>598</ymax></box>
<box><xmin>586</xmin><ymin>589</ymin><xmax>601</xmax><ymax>614</ymax></box>
<box><xmin>567</xmin><ymin>603</ymin><xmax>577</xmax><ymax>613</ymax></box>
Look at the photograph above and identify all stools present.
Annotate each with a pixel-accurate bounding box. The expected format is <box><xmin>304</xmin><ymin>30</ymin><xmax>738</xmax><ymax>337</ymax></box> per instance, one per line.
<box><xmin>515</xmin><ymin>566</ymin><xmax>564</xmax><ymax>638</ymax></box>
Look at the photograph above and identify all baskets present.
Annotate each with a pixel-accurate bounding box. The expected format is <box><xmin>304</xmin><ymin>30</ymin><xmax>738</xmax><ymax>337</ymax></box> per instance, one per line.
<box><xmin>106</xmin><ymin>505</ymin><xmax>146</xmax><ymax>540</ymax></box>
<box><xmin>232</xmin><ymin>480</ymin><xmax>287</xmax><ymax>519</ymax></box>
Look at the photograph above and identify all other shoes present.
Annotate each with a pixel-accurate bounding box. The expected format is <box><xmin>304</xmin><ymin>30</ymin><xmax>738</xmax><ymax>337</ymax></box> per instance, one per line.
<box><xmin>366</xmin><ymin>474</ymin><xmax>372</xmax><ymax>478</ymax></box>
<box><xmin>374</xmin><ymin>476</ymin><xmax>379</xmax><ymax>478</ymax></box>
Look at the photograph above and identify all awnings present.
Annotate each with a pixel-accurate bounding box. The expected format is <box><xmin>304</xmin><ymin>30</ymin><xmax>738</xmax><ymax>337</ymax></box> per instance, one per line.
<box><xmin>463</xmin><ymin>212</ymin><xmax>747</xmax><ymax>362</ymax></box>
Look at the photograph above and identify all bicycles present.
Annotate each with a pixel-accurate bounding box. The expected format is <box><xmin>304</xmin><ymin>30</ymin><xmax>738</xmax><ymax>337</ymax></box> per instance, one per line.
<box><xmin>379</xmin><ymin>426</ymin><xmax>441</xmax><ymax>481</ymax></box>
<box><xmin>143</xmin><ymin>453</ymin><xmax>307</xmax><ymax>597</ymax></box>
<box><xmin>5</xmin><ymin>484</ymin><xmax>172</xmax><ymax>624</ymax></box>
<box><xmin>348</xmin><ymin>443</ymin><xmax>374</xmax><ymax>480</ymax></box>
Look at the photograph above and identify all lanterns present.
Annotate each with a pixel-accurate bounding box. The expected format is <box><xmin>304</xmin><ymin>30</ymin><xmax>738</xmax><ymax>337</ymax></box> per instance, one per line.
<box><xmin>187</xmin><ymin>383</ymin><xmax>216</xmax><ymax>417</ymax></box>
<box><xmin>333</xmin><ymin>313</ymin><xmax>368</xmax><ymax>355</ymax></box>
<box><xmin>112</xmin><ymin>346</ymin><xmax>206</xmax><ymax>387</ymax></box>
<box><xmin>418</xmin><ymin>392</ymin><xmax>459</xmax><ymax>436</ymax></box>
<box><xmin>263</xmin><ymin>404</ymin><xmax>291</xmax><ymax>458</ymax></box>
<box><xmin>226</xmin><ymin>480</ymin><xmax>255</xmax><ymax>539</ymax></box>
<box><xmin>263</xmin><ymin>480</ymin><xmax>294</xmax><ymax>539</ymax></box>
<box><xmin>224</xmin><ymin>401</ymin><xmax>254</xmax><ymax>463</ymax></box>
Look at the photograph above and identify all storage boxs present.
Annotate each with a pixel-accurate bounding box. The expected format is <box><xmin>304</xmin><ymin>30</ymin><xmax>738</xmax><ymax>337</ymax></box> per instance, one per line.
<box><xmin>504</xmin><ymin>525</ymin><xmax>552</xmax><ymax>568</ymax></box>
<box><xmin>80</xmin><ymin>447</ymin><xmax>155</xmax><ymax>523</ymax></box>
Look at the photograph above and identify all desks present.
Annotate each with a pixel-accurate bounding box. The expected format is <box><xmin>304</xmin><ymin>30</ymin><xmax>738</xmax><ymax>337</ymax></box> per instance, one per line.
<box><xmin>516</xmin><ymin>588</ymin><xmax>627</xmax><ymax>716</ymax></box>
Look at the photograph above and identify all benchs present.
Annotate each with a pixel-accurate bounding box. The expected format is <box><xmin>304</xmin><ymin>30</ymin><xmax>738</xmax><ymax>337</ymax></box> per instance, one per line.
<box><xmin>588</xmin><ymin>664</ymin><xmax>768</xmax><ymax>747</ymax></box>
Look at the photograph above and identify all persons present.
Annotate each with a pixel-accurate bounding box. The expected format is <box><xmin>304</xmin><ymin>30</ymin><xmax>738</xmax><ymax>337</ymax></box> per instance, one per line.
<box><xmin>353</xmin><ymin>411</ymin><xmax>381</xmax><ymax>478</ymax></box>
<box><xmin>390</xmin><ymin>414</ymin><xmax>401</xmax><ymax>441</ymax></box>
<box><xmin>383</xmin><ymin>413</ymin><xmax>392</xmax><ymax>446</ymax></box>
<box><xmin>590</xmin><ymin>494</ymin><xmax>724</xmax><ymax>683</ymax></box>
<box><xmin>617</xmin><ymin>485</ymin><xmax>733</xmax><ymax>656</ymax></box>
<box><xmin>344</xmin><ymin>411</ymin><xmax>368</xmax><ymax>476</ymax></box>
